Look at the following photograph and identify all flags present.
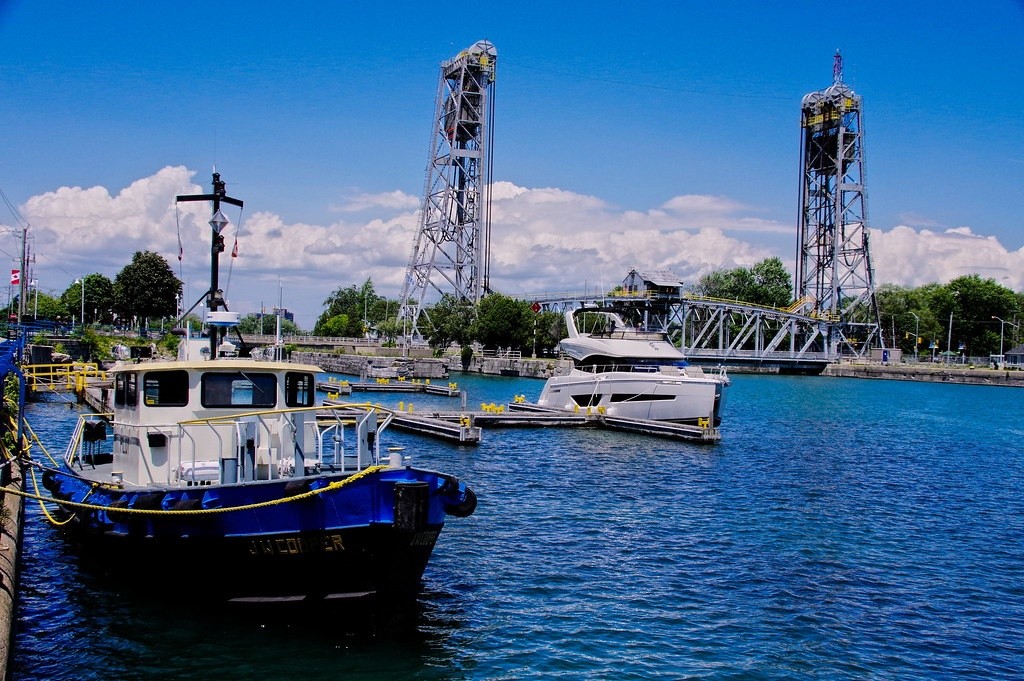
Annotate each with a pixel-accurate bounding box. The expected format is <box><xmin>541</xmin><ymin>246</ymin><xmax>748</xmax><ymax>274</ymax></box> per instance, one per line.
<box><xmin>232</xmin><ymin>240</ymin><xmax>238</xmax><ymax>257</ymax></box>
<box><xmin>11</xmin><ymin>269</ymin><xmax>20</xmax><ymax>284</ymax></box>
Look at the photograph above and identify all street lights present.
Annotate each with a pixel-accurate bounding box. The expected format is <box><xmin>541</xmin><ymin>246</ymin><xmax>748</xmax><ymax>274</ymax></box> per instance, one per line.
<box><xmin>991</xmin><ymin>316</ymin><xmax>1003</xmax><ymax>367</ymax></box>
<box><xmin>225</xmin><ymin>300</ymin><xmax>229</xmax><ymax>337</ymax></box>
<box><xmin>30</xmin><ymin>278</ymin><xmax>39</xmax><ymax>330</ymax></box>
<box><xmin>907</xmin><ymin>312</ymin><xmax>918</xmax><ymax>363</ymax></box>
<box><xmin>175</xmin><ymin>294</ymin><xmax>179</xmax><ymax>328</ymax></box>
<box><xmin>74</xmin><ymin>278</ymin><xmax>84</xmax><ymax>335</ymax></box>
<box><xmin>200</xmin><ymin>300</ymin><xmax>204</xmax><ymax>334</ymax></box>
<box><xmin>1004</xmin><ymin>322</ymin><xmax>1019</xmax><ymax>348</ymax></box>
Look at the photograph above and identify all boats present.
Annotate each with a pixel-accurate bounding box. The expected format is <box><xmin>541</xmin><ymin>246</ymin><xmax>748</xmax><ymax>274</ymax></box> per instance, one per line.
<box><xmin>40</xmin><ymin>162</ymin><xmax>478</xmax><ymax>654</ymax></box>
<box><xmin>536</xmin><ymin>297</ymin><xmax>728</xmax><ymax>442</ymax></box>
